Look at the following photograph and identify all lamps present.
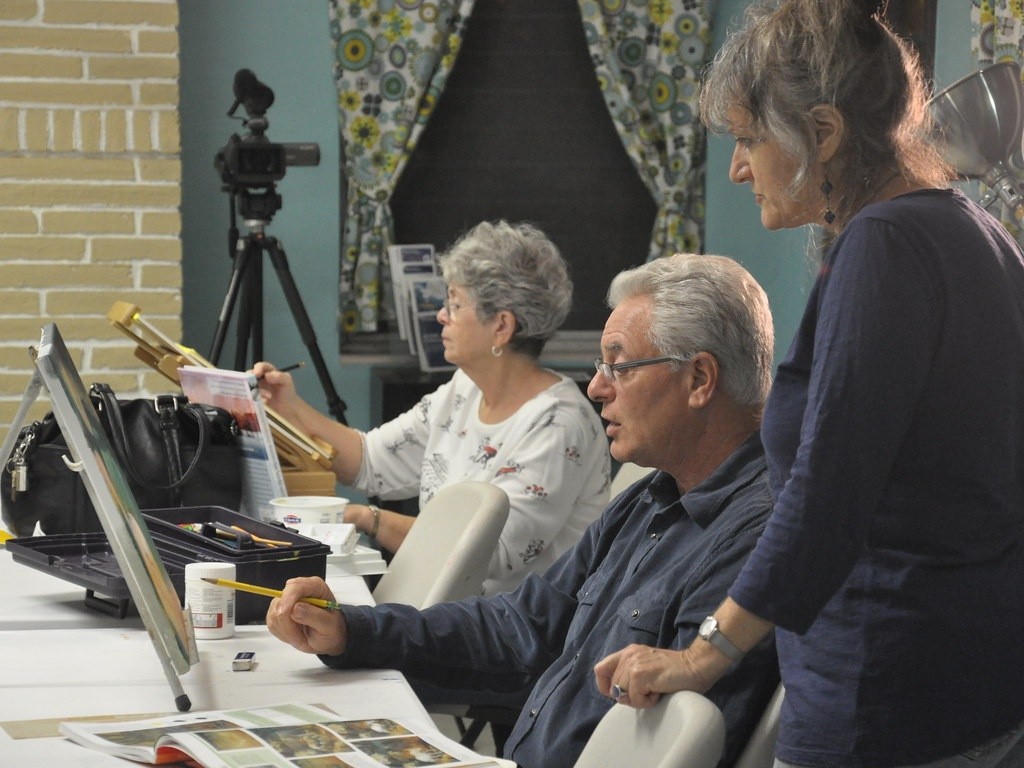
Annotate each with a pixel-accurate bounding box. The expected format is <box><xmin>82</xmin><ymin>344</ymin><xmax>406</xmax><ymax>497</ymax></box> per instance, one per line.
<box><xmin>922</xmin><ymin>60</ymin><xmax>1024</xmax><ymax>231</ymax></box>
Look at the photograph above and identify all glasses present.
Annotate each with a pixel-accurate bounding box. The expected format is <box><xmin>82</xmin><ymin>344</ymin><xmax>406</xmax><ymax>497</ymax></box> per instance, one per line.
<box><xmin>442</xmin><ymin>300</ymin><xmax>485</xmax><ymax>321</ymax></box>
<box><xmin>593</xmin><ymin>350</ymin><xmax>720</xmax><ymax>385</ymax></box>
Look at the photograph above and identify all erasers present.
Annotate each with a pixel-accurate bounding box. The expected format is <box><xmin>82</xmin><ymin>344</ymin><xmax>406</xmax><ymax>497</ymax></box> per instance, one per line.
<box><xmin>232</xmin><ymin>652</ymin><xmax>256</xmax><ymax>671</ymax></box>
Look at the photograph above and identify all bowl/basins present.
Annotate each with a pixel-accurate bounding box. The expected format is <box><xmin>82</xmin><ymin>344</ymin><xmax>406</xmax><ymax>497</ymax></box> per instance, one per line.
<box><xmin>270</xmin><ymin>496</ymin><xmax>349</xmax><ymax>536</ymax></box>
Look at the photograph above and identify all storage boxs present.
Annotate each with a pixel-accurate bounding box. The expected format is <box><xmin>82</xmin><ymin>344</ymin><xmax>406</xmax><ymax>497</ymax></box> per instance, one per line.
<box><xmin>6</xmin><ymin>506</ymin><xmax>333</xmax><ymax>626</ymax></box>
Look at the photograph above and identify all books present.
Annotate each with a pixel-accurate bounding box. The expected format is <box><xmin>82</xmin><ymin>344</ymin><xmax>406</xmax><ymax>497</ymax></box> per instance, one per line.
<box><xmin>387</xmin><ymin>244</ymin><xmax>459</xmax><ymax>372</ymax></box>
<box><xmin>176</xmin><ymin>365</ymin><xmax>288</xmax><ymax>524</ymax></box>
<box><xmin>58</xmin><ymin>700</ymin><xmax>498</xmax><ymax>768</ymax></box>
<box><xmin>285</xmin><ymin>523</ymin><xmax>382</xmax><ymax>564</ymax></box>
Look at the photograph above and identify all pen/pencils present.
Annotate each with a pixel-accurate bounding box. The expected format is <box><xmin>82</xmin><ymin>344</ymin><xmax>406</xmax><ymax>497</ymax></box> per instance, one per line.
<box><xmin>230</xmin><ymin>525</ymin><xmax>278</xmax><ymax>549</ymax></box>
<box><xmin>256</xmin><ymin>362</ymin><xmax>304</xmax><ymax>382</ymax></box>
<box><xmin>215</xmin><ymin>529</ymin><xmax>293</xmax><ymax>546</ymax></box>
<box><xmin>201</xmin><ymin>577</ymin><xmax>338</xmax><ymax>610</ymax></box>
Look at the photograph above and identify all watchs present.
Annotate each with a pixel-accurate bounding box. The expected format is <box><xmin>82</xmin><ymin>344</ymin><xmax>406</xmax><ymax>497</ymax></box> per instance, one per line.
<box><xmin>364</xmin><ymin>505</ymin><xmax>380</xmax><ymax>539</ymax></box>
<box><xmin>698</xmin><ymin>616</ymin><xmax>745</xmax><ymax>665</ymax></box>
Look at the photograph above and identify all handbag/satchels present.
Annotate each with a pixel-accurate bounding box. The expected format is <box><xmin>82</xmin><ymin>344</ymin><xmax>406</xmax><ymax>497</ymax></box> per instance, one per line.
<box><xmin>1</xmin><ymin>381</ymin><xmax>246</xmax><ymax>536</ymax></box>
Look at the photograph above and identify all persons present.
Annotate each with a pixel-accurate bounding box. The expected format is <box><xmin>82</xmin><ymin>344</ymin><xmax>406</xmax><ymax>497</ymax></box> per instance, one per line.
<box><xmin>267</xmin><ymin>253</ymin><xmax>780</xmax><ymax>768</ymax></box>
<box><xmin>594</xmin><ymin>0</ymin><xmax>1024</xmax><ymax>768</ymax></box>
<box><xmin>246</xmin><ymin>219</ymin><xmax>612</xmax><ymax>598</ymax></box>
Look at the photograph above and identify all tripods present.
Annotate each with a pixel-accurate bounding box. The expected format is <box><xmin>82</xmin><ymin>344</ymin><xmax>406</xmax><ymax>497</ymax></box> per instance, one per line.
<box><xmin>200</xmin><ymin>183</ymin><xmax>352</xmax><ymax>431</ymax></box>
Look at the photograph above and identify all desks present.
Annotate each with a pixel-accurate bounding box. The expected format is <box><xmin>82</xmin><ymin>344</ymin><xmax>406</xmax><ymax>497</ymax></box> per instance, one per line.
<box><xmin>0</xmin><ymin>549</ymin><xmax>437</xmax><ymax>768</ymax></box>
<box><xmin>369</xmin><ymin>365</ymin><xmax>604</xmax><ymax>507</ymax></box>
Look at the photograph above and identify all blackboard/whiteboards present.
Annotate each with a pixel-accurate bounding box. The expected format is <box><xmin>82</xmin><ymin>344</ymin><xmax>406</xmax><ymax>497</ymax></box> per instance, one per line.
<box><xmin>339</xmin><ymin>0</ymin><xmax>660</xmax><ymax>363</ymax></box>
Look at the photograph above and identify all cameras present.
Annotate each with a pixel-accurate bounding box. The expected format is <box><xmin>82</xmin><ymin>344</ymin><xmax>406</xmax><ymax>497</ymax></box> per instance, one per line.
<box><xmin>213</xmin><ymin>129</ymin><xmax>322</xmax><ymax>185</ymax></box>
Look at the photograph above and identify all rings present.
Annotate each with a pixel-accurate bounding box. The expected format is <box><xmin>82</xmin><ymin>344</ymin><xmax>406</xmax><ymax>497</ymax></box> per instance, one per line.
<box><xmin>607</xmin><ymin>685</ymin><xmax>627</xmax><ymax>701</ymax></box>
